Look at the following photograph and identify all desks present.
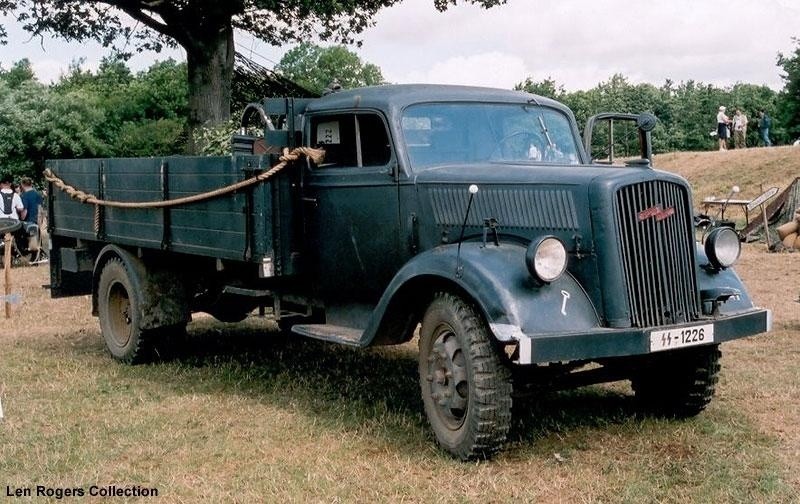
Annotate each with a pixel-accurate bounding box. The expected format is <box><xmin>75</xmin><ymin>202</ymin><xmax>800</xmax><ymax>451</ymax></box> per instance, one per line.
<box><xmin>701</xmin><ymin>199</ymin><xmax>751</xmax><ymax>226</ymax></box>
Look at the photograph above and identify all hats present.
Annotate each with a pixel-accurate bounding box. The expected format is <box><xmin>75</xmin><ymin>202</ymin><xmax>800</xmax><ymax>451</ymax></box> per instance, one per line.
<box><xmin>719</xmin><ymin>106</ymin><xmax>726</xmax><ymax>110</ymax></box>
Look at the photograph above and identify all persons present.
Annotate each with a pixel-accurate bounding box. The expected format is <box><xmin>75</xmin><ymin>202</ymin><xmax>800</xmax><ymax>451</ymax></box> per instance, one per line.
<box><xmin>717</xmin><ymin>105</ymin><xmax>733</xmax><ymax>151</ymax></box>
<box><xmin>731</xmin><ymin>108</ymin><xmax>749</xmax><ymax>149</ymax></box>
<box><xmin>0</xmin><ymin>176</ymin><xmax>45</xmax><ymax>268</ymax></box>
<box><xmin>756</xmin><ymin>109</ymin><xmax>771</xmax><ymax>147</ymax></box>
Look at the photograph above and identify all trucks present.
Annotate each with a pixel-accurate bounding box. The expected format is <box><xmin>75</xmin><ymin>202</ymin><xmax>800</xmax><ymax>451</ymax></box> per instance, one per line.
<box><xmin>45</xmin><ymin>82</ymin><xmax>773</xmax><ymax>464</ymax></box>
